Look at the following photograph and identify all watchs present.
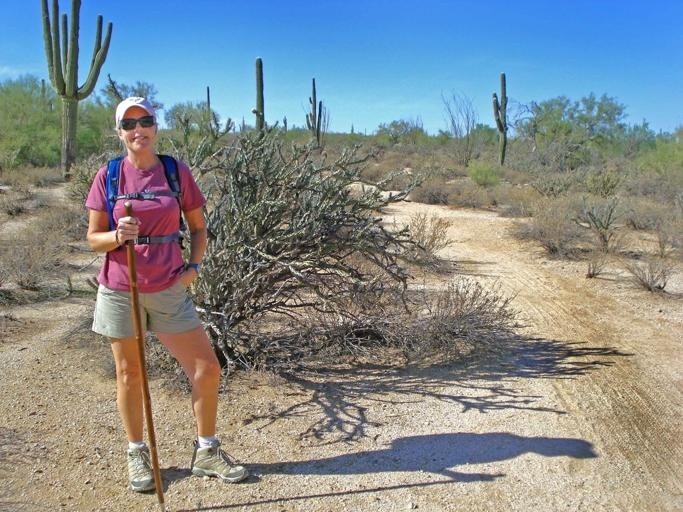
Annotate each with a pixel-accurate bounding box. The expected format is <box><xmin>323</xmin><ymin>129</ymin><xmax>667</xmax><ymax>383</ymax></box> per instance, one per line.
<box><xmin>188</xmin><ymin>263</ymin><xmax>203</xmax><ymax>273</ymax></box>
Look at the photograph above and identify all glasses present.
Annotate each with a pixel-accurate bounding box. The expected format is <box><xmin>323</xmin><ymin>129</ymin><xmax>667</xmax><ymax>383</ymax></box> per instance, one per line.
<box><xmin>119</xmin><ymin>117</ymin><xmax>154</xmax><ymax>131</ymax></box>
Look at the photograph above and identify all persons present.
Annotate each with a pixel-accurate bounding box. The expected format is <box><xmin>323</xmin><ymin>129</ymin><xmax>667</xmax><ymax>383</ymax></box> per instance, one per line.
<box><xmin>85</xmin><ymin>95</ymin><xmax>250</xmax><ymax>491</ymax></box>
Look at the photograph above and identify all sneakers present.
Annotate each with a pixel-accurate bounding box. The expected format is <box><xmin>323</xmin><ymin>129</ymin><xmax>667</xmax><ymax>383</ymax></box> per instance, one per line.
<box><xmin>125</xmin><ymin>446</ymin><xmax>156</xmax><ymax>493</ymax></box>
<box><xmin>191</xmin><ymin>445</ymin><xmax>249</xmax><ymax>482</ymax></box>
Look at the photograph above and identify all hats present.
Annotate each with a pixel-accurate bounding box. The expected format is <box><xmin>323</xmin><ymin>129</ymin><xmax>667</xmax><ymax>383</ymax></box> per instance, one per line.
<box><xmin>114</xmin><ymin>96</ymin><xmax>156</xmax><ymax>128</ymax></box>
